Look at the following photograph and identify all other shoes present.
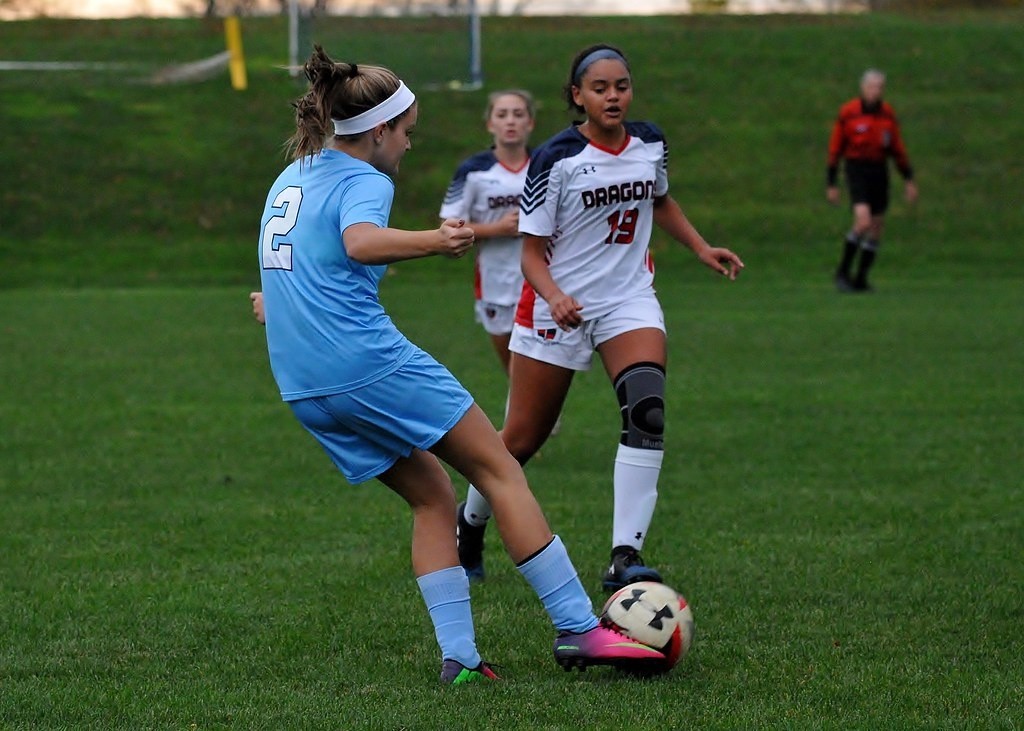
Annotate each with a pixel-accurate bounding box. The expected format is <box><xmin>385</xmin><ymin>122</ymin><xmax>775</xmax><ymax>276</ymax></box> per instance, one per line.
<box><xmin>853</xmin><ymin>279</ymin><xmax>873</xmax><ymax>291</ymax></box>
<box><xmin>837</xmin><ymin>271</ymin><xmax>850</xmax><ymax>292</ymax></box>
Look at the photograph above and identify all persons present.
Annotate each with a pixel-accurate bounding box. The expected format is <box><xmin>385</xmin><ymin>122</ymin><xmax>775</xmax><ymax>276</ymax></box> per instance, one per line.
<box><xmin>825</xmin><ymin>66</ymin><xmax>919</xmax><ymax>293</ymax></box>
<box><xmin>437</xmin><ymin>86</ymin><xmax>563</xmax><ymax>459</ymax></box>
<box><xmin>248</xmin><ymin>43</ymin><xmax>670</xmax><ymax>685</ymax></box>
<box><xmin>455</xmin><ymin>42</ymin><xmax>745</xmax><ymax>593</ymax></box>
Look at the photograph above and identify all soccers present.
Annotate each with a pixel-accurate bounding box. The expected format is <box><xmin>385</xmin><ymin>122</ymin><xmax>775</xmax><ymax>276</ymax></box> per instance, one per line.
<box><xmin>599</xmin><ymin>580</ymin><xmax>695</xmax><ymax>670</ymax></box>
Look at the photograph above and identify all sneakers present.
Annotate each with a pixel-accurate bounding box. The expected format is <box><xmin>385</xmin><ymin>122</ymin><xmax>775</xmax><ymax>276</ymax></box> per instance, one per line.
<box><xmin>553</xmin><ymin>623</ymin><xmax>665</xmax><ymax>672</ymax></box>
<box><xmin>455</xmin><ymin>501</ymin><xmax>488</xmax><ymax>573</ymax></box>
<box><xmin>602</xmin><ymin>546</ymin><xmax>663</xmax><ymax>592</ymax></box>
<box><xmin>439</xmin><ymin>658</ymin><xmax>507</xmax><ymax>685</ymax></box>
